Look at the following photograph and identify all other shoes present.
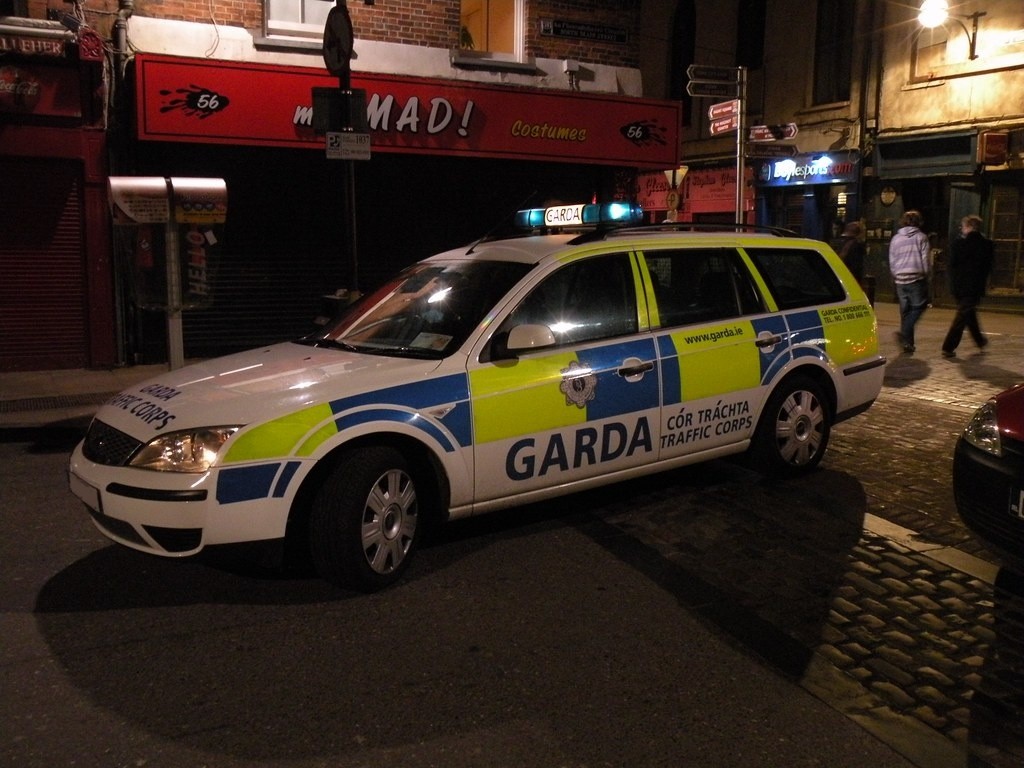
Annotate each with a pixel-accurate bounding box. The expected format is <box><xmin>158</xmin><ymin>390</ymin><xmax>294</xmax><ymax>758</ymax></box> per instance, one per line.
<box><xmin>941</xmin><ymin>351</ymin><xmax>956</xmax><ymax>357</ymax></box>
<box><xmin>903</xmin><ymin>344</ymin><xmax>916</xmax><ymax>353</ymax></box>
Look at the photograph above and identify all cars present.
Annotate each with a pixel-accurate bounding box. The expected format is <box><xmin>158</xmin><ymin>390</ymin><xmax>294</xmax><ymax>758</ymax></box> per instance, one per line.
<box><xmin>68</xmin><ymin>200</ymin><xmax>887</xmax><ymax>585</ymax></box>
<box><xmin>952</xmin><ymin>380</ymin><xmax>1024</xmax><ymax>546</ymax></box>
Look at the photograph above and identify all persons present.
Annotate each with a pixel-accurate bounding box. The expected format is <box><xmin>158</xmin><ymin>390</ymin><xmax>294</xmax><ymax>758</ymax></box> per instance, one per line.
<box><xmin>941</xmin><ymin>214</ymin><xmax>996</xmax><ymax>357</ymax></box>
<box><xmin>889</xmin><ymin>211</ymin><xmax>930</xmax><ymax>353</ymax></box>
<box><xmin>833</xmin><ymin>222</ymin><xmax>868</xmax><ymax>289</ymax></box>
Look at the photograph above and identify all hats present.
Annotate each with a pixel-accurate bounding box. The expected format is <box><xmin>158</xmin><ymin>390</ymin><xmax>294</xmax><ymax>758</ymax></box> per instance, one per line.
<box><xmin>841</xmin><ymin>222</ymin><xmax>860</xmax><ymax>236</ymax></box>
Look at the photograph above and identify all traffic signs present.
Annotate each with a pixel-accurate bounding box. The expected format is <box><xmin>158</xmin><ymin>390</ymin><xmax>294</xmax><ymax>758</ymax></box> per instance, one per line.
<box><xmin>685</xmin><ymin>64</ymin><xmax>799</xmax><ymax>142</ymax></box>
